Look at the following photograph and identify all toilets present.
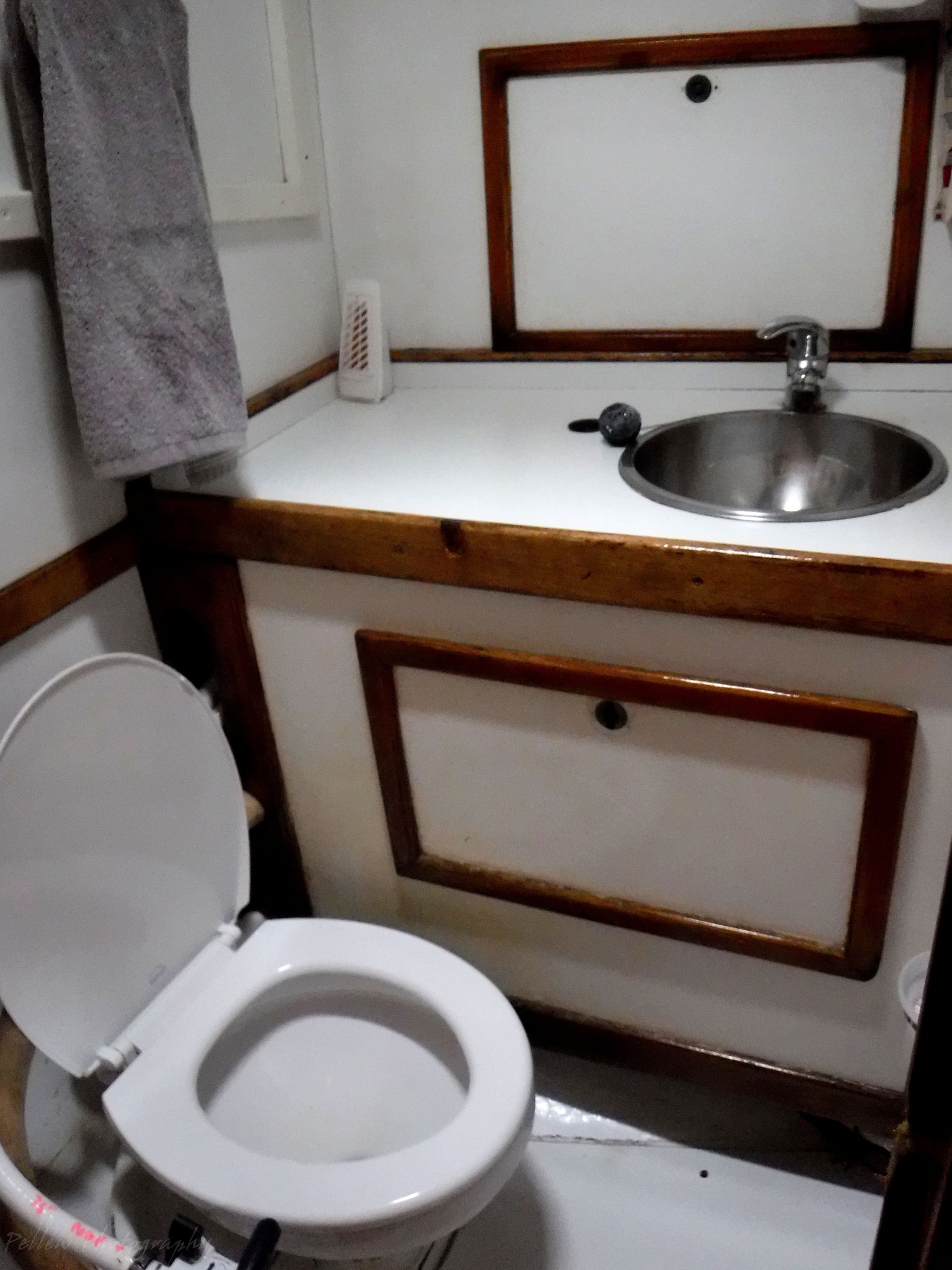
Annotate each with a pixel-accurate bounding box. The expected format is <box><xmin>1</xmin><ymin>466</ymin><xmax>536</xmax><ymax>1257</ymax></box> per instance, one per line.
<box><xmin>0</xmin><ymin>649</ymin><xmax>534</xmax><ymax>1262</ymax></box>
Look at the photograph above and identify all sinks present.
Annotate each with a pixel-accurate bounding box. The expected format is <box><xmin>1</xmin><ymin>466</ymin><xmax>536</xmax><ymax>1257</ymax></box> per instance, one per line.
<box><xmin>618</xmin><ymin>409</ymin><xmax>948</xmax><ymax>522</ymax></box>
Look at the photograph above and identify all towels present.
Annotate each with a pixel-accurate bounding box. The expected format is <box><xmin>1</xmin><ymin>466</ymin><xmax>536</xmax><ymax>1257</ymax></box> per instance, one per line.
<box><xmin>0</xmin><ymin>1</ymin><xmax>249</xmax><ymax>494</ymax></box>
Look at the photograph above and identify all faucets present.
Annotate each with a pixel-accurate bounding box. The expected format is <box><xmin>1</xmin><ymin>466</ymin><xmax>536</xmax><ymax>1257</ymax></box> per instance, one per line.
<box><xmin>757</xmin><ymin>316</ymin><xmax>829</xmax><ymax>412</ymax></box>
